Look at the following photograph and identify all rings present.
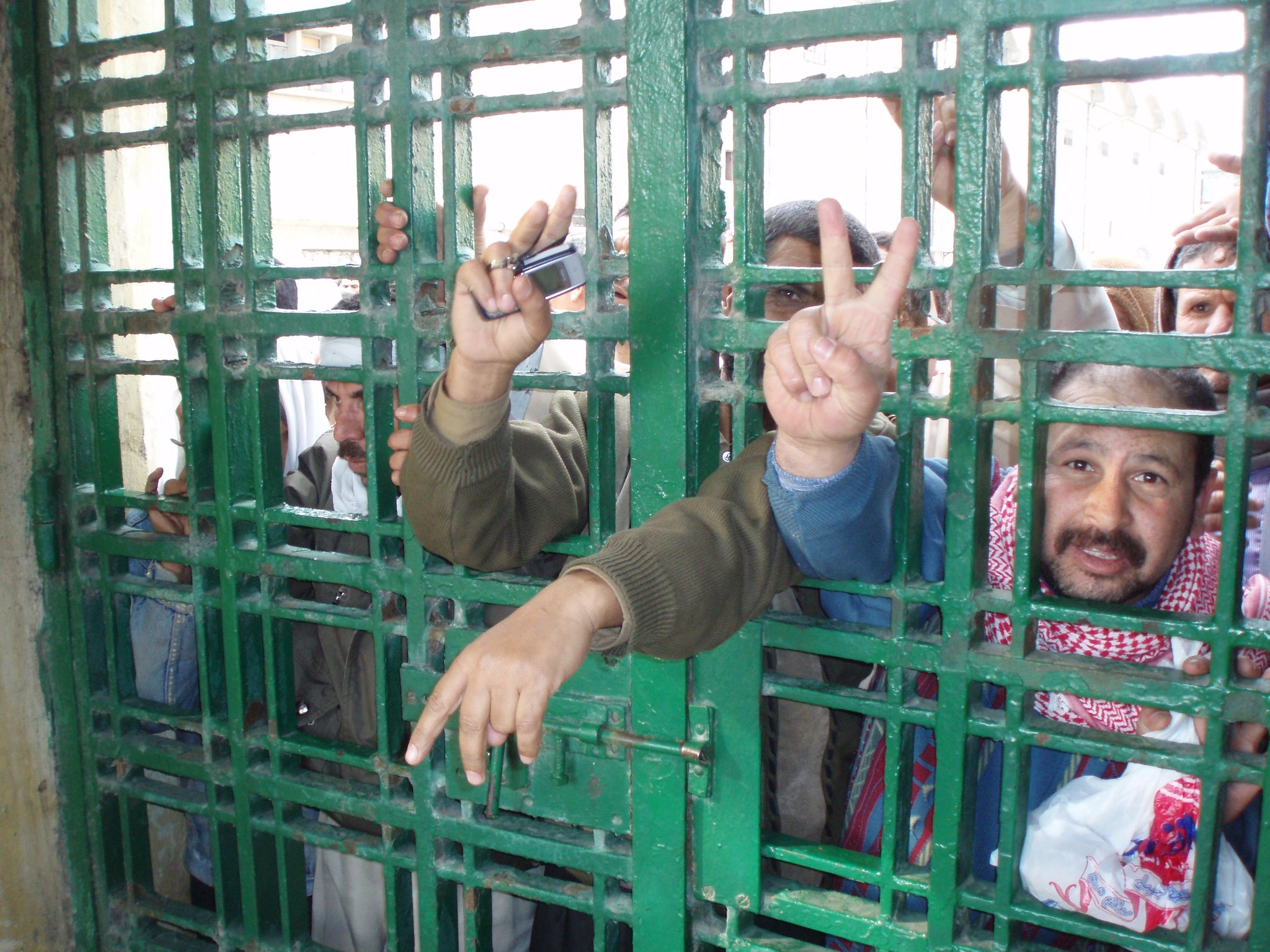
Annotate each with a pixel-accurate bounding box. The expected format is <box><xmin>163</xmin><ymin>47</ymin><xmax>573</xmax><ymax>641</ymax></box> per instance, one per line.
<box><xmin>486</xmin><ymin>257</ymin><xmax>518</xmax><ymax>272</ymax></box>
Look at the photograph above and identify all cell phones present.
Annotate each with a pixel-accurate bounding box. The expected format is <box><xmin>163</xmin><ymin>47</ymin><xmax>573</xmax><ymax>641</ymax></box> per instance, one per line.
<box><xmin>470</xmin><ymin>243</ymin><xmax>585</xmax><ymax>321</ymax></box>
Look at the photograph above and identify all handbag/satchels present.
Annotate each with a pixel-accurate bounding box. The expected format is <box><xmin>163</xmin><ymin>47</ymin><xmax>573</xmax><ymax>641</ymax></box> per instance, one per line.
<box><xmin>989</xmin><ymin>638</ymin><xmax>1256</xmax><ymax>944</ymax></box>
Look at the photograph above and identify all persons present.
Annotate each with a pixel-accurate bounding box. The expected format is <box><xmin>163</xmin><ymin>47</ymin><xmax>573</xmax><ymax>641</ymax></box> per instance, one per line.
<box><xmin>116</xmin><ymin>46</ymin><xmax>1270</xmax><ymax>952</ymax></box>
